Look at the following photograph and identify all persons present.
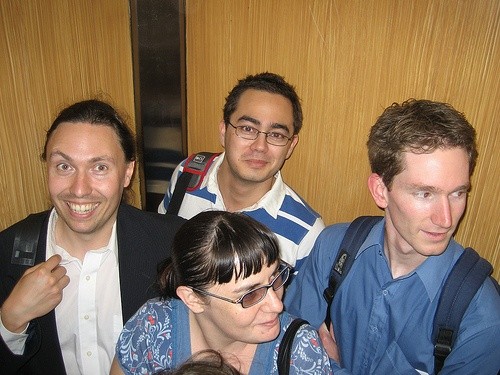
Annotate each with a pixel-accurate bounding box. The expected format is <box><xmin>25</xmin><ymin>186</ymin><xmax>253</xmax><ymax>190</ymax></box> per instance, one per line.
<box><xmin>158</xmin><ymin>72</ymin><xmax>327</xmax><ymax>288</ymax></box>
<box><xmin>109</xmin><ymin>210</ymin><xmax>333</xmax><ymax>375</ymax></box>
<box><xmin>0</xmin><ymin>99</ymin><xmax>189</xmax><ymax>375</ymax></box>
<box><xmin>282</xmin><ymin>98</ymin><xmax>500</xmax><ymax>375</ymax></box>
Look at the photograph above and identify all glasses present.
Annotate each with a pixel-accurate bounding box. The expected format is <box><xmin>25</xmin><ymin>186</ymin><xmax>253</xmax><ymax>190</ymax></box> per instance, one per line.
<box><xmin>185</xmin><ymin>258</ymin><xmax>290</xmax><ymax>308</ymax></box>
<box><xmin>228</xmin><ymin>120</ymin><xmax>295</xmax><ymax>146</ymax></box>
